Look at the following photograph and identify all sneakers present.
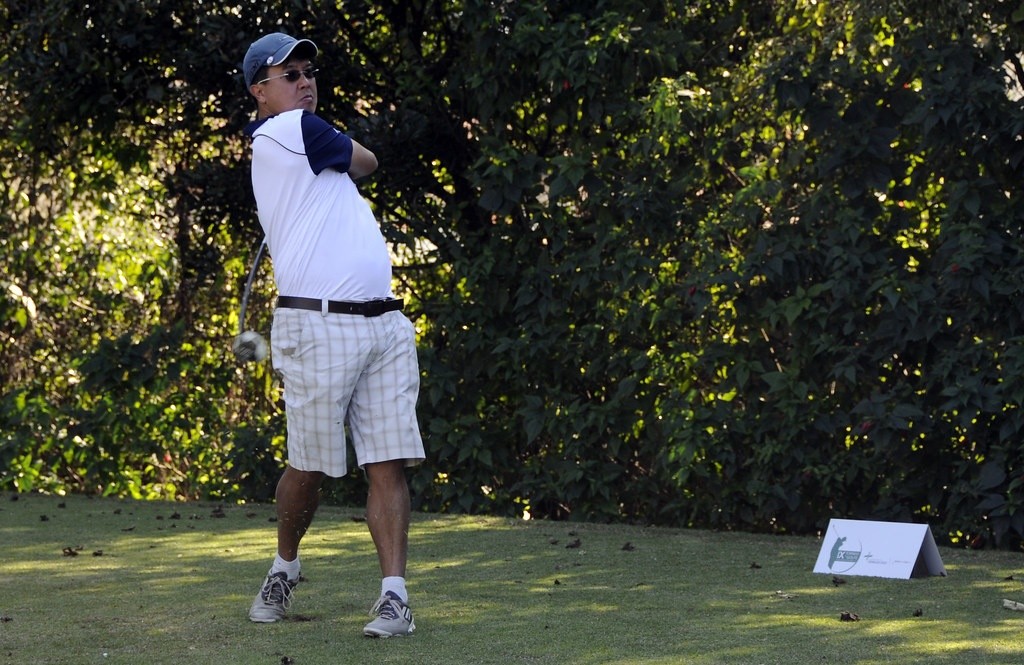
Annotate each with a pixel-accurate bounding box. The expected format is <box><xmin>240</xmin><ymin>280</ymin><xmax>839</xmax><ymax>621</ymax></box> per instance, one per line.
<box><xmin>363</xmin><ymin>591</ymin><xmax>417</xmax><ymax>639</ymax></box>
<box><xmin>249</xmin><ymin>566</ymin><xmax>301</xmax><ymax>622</ymax></box>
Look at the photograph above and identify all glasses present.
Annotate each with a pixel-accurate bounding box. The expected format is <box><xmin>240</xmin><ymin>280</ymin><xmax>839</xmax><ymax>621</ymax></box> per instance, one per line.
<box><xmin>257</xmin><ymin>65</ymin><xmax>318</xmax><ymax>84</ymax></box>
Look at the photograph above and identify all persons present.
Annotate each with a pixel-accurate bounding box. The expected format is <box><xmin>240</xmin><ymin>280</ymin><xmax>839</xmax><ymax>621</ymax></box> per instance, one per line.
<box><xmin>242</xmin><ymin>31</ymin><xmax>427</xmax><ymax>637</ymax></box>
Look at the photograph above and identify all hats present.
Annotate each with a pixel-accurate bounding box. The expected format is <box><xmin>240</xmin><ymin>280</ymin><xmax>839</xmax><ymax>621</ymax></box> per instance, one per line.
<box><xmin>242</xmin><ymin>31</ymin><xmax>319</xmax><ymax>90</ymax></box>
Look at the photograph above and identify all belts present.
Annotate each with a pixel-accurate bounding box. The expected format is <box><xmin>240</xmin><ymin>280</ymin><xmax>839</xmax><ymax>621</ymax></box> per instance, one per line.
<box><xmin>277</xmin><ymin>294</ymin><xmax>404</xmax><ymax>317</ymax></box>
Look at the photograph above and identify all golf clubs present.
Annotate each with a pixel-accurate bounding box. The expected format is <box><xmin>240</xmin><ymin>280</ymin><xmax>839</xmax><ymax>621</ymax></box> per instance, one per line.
<box><xmin>232</xmin><ymin>237</ymin><xmax>272</xmax><ymax>365</ymax></box>
